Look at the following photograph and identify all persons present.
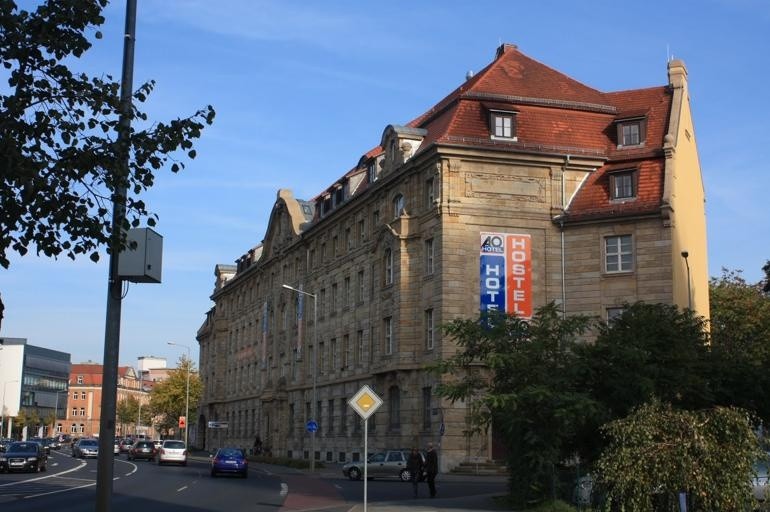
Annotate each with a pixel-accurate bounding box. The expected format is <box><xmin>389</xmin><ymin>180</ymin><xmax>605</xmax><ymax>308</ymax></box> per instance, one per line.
<box><xmin>422</xmin><ymin>441</ymin><xmax>439</xmax><ymax>499</ymax></box>
<box><xmin>406</xmin><ymin>444</ymin><xmax>424</xmax><ymax>500</ymax></box>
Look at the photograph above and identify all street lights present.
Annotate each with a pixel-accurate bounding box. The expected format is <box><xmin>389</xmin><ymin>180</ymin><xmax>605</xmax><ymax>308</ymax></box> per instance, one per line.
<box><xmin>283</xmin><ymin>284</ymin><xmax>318</xmax><ymax>470</ymax></box>
<box><xmin>682</xmin><ymin>252</ymin><xmax>691</xmax><ymax>312</ymax></box>
<box><xmin>169</xmin><ymin>342</ymin><xmax>190</xmax><ymax>455</ymax></box>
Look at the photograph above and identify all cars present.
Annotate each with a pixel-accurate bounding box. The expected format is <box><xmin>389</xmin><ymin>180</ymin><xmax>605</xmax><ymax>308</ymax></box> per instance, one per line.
<box><xmin>114</xmin><ymin>436</ymin><xmax>162</xmax><ymax>461</ymax></box>
<box><xmin>210</xmin><ymin>447</ymin><xmax>248</xmax><ymax>478</ymax></box>
<box><xmin>158</xmin><ymin>440</ymin><xmax>188</xmax><ymax>467</ymax></box>
<box><xmin>73</xmin><ymin>439</ymin><xmax>99</xmax><ymax>458</ymax></box>
<box><xmin>30</xmin><ymin>434</ymin><xmax>72</xmax><ymax>456</ymax></box>
<box><xmin>0</xmin><ymin>442</ymin><xmax>47</xmax><ymax>474</ymax></box>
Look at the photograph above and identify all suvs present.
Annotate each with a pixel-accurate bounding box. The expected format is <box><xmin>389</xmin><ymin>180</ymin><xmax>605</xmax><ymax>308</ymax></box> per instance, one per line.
<box><xmin>343</xmin><ymin>452</ymin><xmax>428</xmax><ymax>483</ymax></box>
